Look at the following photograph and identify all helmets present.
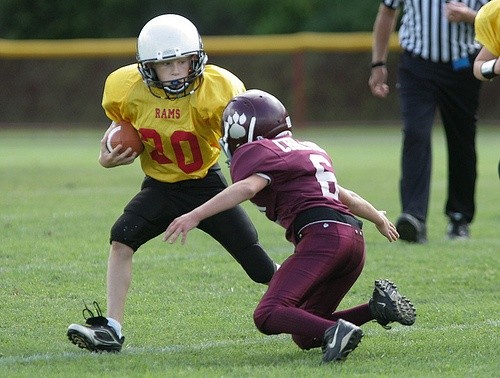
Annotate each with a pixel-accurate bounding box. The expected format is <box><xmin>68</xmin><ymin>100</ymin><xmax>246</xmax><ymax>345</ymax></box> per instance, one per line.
<box><xmin>218</xmin><ymin>90</ymin><xmax>294</xmax><ymax>169</ymax></box>
<box><xmin>136</xmin><ymin>13</ymin><xmax>208</xmax><ymax>100</ymax></box>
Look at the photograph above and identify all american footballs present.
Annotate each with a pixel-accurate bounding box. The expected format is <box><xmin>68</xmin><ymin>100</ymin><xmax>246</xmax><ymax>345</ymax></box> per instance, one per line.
<box><xmin>105</xmin><ymin>121</ymin><xmax>145</xmax><ymax>158</ymax></box>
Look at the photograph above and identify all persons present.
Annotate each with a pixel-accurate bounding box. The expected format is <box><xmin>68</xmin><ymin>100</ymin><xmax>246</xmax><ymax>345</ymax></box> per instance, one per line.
<box><xmin>162</xmin><ymin>89</ymin><xmax>416</xmax><ymax>363</ymax></box>
<box><xmin>369</xmin><ymin>0</ymin><xmax>491</xmax><ymax>245</ymax></box>
<box><xmin>473</xmin><ymin>0</ymin><xmax>500</xmax><ymax>179</ymax></box>
<box><xmin>67</xmin><ymin>15</ymin><xmax>280</xmax><ymax>354</ymax></box>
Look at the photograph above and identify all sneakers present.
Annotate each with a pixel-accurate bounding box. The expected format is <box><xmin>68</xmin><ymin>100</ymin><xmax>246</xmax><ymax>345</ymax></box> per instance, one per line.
<box><xmin>446</xmin><ymin>212</ymin><xmax>469</xmax><ymax>237</ymax></box>
<box><xmin>395</xmin><ymin>213</ymin><xmax>427</xmax><ymax>245</ymax></box>
<box><xmin>66</xmin><ymin>301</ymin><xmax>125</xmax><ymax>352</ymax></box>
<box><xmin>320</xmin><ymin>320</ymin><xmax>363</xmax><ymax>364</ymax></box>
<box><xmin>368</xmin><ymin>280</ymin><xmax>416</xmax><ymax>329</ymax></box>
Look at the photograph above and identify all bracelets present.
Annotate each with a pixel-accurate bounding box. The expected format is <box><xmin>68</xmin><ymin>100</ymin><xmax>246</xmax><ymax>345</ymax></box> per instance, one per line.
<box><xmin>368</xmin><ymin>61</ymin><xmax>386</xmax><ymax>69</ymax></box>
<box><xmin>481</xmin><ymin>57</ymin><xmax>500</xmax><ymax>80</ymax></box>
